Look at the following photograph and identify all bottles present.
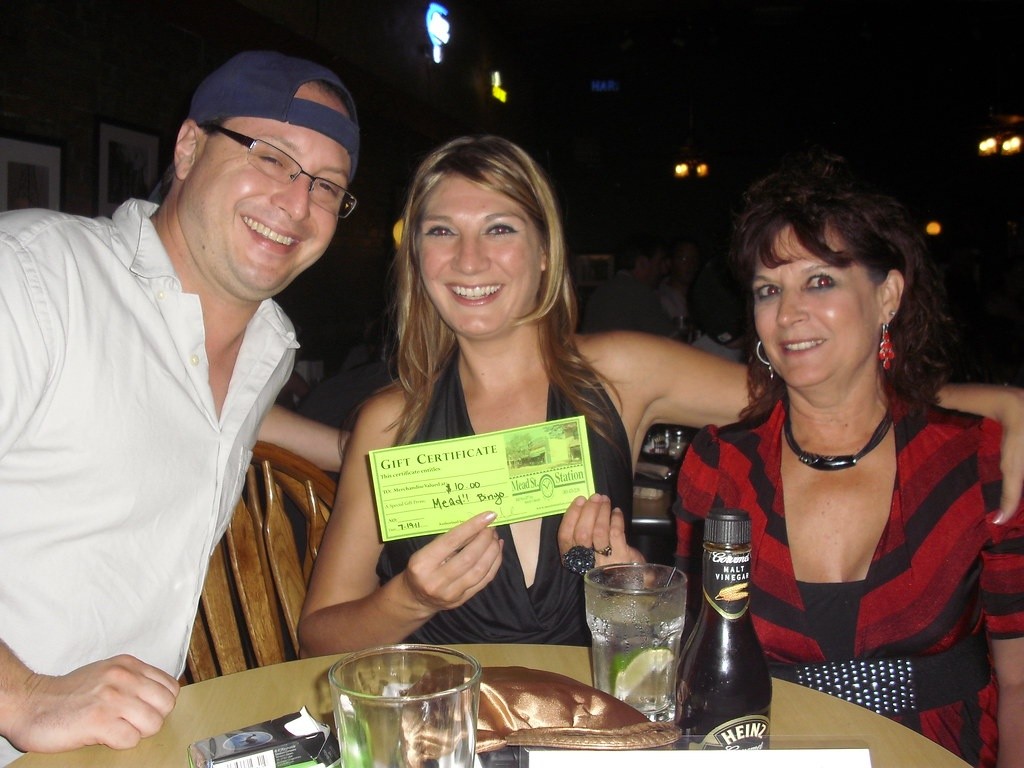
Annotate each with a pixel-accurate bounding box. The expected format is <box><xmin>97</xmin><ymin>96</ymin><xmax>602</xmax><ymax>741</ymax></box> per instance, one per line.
<box><xmin>676</xmin><ymin>506</ymin><xmax>773</xmax><ymax>750</ymax></box>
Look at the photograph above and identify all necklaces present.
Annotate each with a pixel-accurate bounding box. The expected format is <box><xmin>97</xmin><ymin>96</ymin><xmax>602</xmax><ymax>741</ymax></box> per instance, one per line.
<box><xmin>783</xmin><ymin>403</ymin><xmax>891</xmax><ymax>471</ymax></box>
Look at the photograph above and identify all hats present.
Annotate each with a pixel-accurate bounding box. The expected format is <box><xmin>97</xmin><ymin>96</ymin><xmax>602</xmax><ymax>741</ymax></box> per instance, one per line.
<box><xmin>148</xmin><ymin>50</ymin><xmax>362</xmax><ymax>212</ymax></box>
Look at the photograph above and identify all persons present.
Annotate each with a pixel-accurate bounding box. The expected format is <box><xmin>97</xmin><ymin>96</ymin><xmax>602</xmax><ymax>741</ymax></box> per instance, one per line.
<box><xmin>0</xmin><ymin>51</ymin><xmax>361</xmax><ymax>768</ymax></box>
<box><xmin>273</xmin><ymin>287</ymin><xmax>401</xmax><ymax>430</ymax></box>
<box><xmin>298</xmin><ymin>133</ymin><xmax>1024</xmax><ymax>657</ymax></box>
<box><xmin>558</xmin><ymin>156</ymin><xmax>1024</xmax><ymax>768</ymax></box>
<box><xmin>580</xmin><ymin>236</ymin><xmax>752</xmax><ymax>351</ymax></box>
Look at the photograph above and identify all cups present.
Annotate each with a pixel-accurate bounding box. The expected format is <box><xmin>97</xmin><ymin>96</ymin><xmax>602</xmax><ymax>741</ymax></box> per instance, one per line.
<box><xmin>674</xmin><ymin>316</ymin><xmax>693</xmax><ymax>344</ymax></box>
<box><xmin>585</xmin><ymin>563</ymin><xmax>687</xmax><ymax>723</ymax></box>
<box><xmin>328</xmin><ymin>644</ymin><xmax>482</xmax><ymax>768</ymax></box>
<box><xmin>650</xmin><ymin>427</ymin><xmax>687</xmax><ymax>458</ymax></box>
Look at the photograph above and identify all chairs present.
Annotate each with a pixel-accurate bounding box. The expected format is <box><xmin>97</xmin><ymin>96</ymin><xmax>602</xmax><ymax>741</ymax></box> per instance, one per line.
<box><xmin>176</xmin><ymin>440</ymin><xmax>338</xmax><ymax>693</ymax></box>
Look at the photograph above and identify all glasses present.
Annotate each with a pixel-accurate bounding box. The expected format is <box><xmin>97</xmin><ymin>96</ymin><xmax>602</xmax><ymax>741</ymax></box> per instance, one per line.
<box><xmin>197</xmin><ymin>122</ymin><xmax>358</xmax><ymax>219</ymax></box>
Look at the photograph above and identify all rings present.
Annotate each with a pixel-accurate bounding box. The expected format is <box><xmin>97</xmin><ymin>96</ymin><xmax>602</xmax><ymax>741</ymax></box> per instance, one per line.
<box><xmin>593</xmin><ymin>545</ymin><xmax>612</xmax><ymax>556</ymax></box>
<box><xmin>563</xmin><ymin>545</ymin><xmax>596</xmax><ymax>574</ymax></box>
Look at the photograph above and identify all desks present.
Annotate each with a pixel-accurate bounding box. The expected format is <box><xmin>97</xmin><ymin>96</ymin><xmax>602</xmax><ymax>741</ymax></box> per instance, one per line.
<box><xmin>2</xmin><ymin>640</ymin><xmax>980</xmax><ymax>768</ymax></box>
